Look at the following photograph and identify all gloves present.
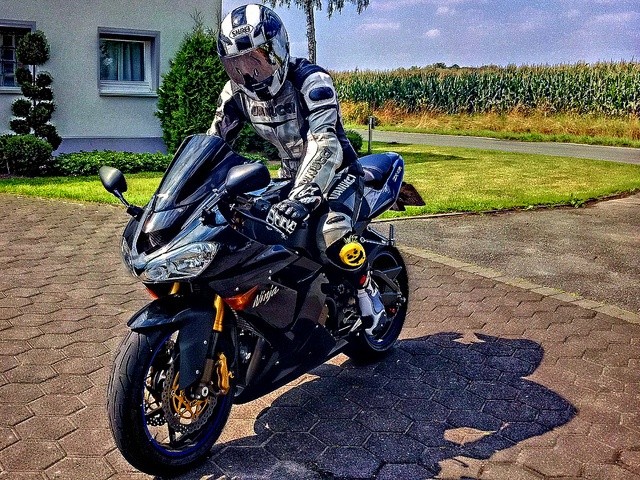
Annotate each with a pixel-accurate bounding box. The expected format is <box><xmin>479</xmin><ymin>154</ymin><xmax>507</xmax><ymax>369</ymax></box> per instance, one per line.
<box><xmin>265</xmin><ymin>199</ymin><xmax>311</xmax><ymax>241</ymax></box>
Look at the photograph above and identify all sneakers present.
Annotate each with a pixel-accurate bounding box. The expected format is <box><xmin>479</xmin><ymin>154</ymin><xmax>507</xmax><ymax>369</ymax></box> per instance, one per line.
<box><xmin>355</xmin><ymin>267</ymin><xmax>389</xmax><ymax>340</ymax></box>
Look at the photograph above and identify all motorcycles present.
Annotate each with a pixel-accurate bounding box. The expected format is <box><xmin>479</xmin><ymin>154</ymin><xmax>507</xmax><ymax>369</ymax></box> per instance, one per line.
<box><xmin>99</xmin><ymin>133</ymin><xmax>426</xmax><ymax>477</ymax></box>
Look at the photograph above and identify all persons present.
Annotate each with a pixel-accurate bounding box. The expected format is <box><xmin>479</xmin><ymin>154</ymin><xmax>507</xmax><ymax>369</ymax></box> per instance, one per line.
<box><xmin>205</xmin><ymin>2</ymin><xmax>389</xmax><ymax>337</ymax></box>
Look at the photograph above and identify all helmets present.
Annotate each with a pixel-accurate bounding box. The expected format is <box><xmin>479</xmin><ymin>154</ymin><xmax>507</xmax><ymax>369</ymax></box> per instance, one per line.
<box><xmin>218</xmin><ymin>3</ymin><xmax>290</xmax><ymax>103</ymax></box>
